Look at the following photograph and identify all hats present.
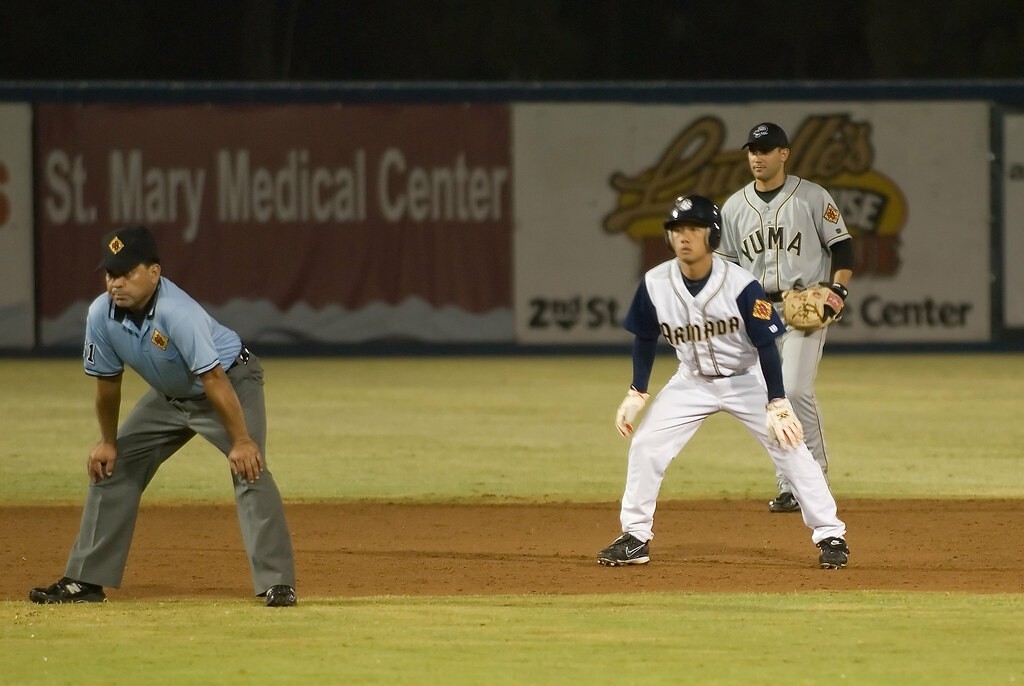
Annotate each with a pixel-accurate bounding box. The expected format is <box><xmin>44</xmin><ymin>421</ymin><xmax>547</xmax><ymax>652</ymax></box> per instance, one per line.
<box><xmin>741</xmin><ymin>122</ymin><xmax>788</xmax><ymax>153</ymax></box>
<box><xmin>93</xmin><ymin>225</ymin><xmax>156</xmax><ymax>276</ymax></box>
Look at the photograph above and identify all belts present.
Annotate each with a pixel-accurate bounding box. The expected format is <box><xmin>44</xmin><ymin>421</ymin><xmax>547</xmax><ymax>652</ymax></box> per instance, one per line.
<box><xmin>765</xmin><ymin>291</ymin><xmax>784</xmax><ymax>303</ymax></box>
<box><xmin>229</xmin><ymin>343</ymin><xmax>250</xmax><ymax>369</ymax></box>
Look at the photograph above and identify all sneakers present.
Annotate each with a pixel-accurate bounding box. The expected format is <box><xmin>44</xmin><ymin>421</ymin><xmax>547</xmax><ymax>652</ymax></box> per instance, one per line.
<box><xmin>29</xmin><ymin>577</ymin><xmax>107</xmax><ymax>604</ymax></box>
<box><xmin>597</xmin><ymin>532</ymin><xmax>650</xmax><ymax>566</ymax></box>
<box><xmin>266</xmin><ymin>585</ymin><xmax>296</xmax><ymax>605</ymax></box>
<box><xmin>768</xmin><ymin>493</ymin><xmax>801</xmax><ymax>513</ymax></box>
<box><xmin>816</xmin><ymin>537</ymin><xmax>850</xmax><ymax>570</ymax></box>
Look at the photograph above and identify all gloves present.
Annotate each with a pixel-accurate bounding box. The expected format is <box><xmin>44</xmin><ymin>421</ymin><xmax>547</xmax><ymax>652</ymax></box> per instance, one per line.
<box><xmin>766</xmin><ymin>398</ymin><xmax>803</xmax><ymax>450</ymax></box>
<box><xmin>614</xmin><ymin>388</ymin><xmax>650</xmax><ymax>438</ymax></box>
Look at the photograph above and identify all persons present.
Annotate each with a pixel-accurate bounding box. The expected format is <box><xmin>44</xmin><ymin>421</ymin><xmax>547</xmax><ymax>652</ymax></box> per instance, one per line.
<box><xmin>714</xmin><ymin>122</ymin><xmax>852</xmax><ymax>512</ymax></box>
<box><xmin>29</xmin><ymin>227</ymin><xmax>297</xmax><ymax>607</ymax></box>
<box><xmin>598</xmin><ymin>193</ymin><xmax>848</xmax><ymax>571</ymax></box>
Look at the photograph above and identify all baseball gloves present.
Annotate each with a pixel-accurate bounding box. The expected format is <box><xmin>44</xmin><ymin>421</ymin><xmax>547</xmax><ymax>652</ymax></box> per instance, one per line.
<box><xmin>781</xmin><ymin>286</ymin><xmax>844</xmax><ymax>330</ymax></box>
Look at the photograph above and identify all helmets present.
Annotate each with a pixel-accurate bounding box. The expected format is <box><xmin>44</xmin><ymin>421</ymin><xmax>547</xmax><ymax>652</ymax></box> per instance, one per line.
<box><xmin>663</xmin><ymin>194</ymin><xmax>722</xmax><ymax>254</ymax></box>
